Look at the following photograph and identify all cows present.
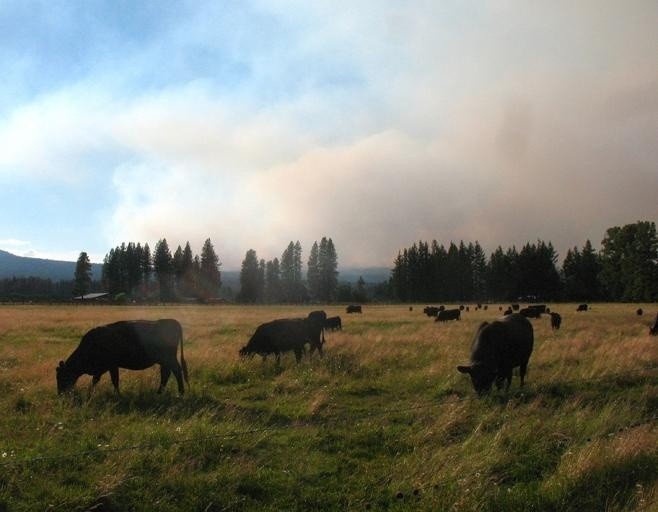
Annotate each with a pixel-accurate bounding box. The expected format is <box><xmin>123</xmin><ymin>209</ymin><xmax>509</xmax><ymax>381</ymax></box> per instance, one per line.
<box><xmin>548</xmin><ymin>312</ymin><xmax>561</xmax><ymax>330</ymax></box>
<box><xmin>307</xmin><ymin>309</ymin><xmax>343</xmax><ymax>332</ymax></box>
<box><xmin>423</xmin><ymin>299</ymin><xmax>552</xmax><ymax>323</ymax></box>
<box><xmin>637</xmin><ymin>308</ymin><xmax>643</xmax><ymax>316</ymax></box>
<box><xmin>409</xmin><ymin>306</ymin><xmax>413</xmax><ymax>311</ymax></box>
<box><xmin>576</xmin><ymin>303</ymin><xmax>588</xmax><ymax>311</ymax></box>
<box><xmin>346</xmin><ymin>304</ymin><xmax>362</xmax><ymax>318</ymax></box>
<box><xmin>649</xmin><ymin>314</ymin><xmax>658</xmax><ymax>336</ymax></box>
<box><xmin>238</xmin><ymin>317</ymin><xmax>324</xmax><ymax>368</ymax></box>
<box><xmin>456</xmin><ymin>312</ymin><xmax>533</xmax><ymax>397</ymax></box>
<box><xmin>54</xmin><ymin>316</ymin><xmax>191</xmax><ymax>400</ymax></box>
